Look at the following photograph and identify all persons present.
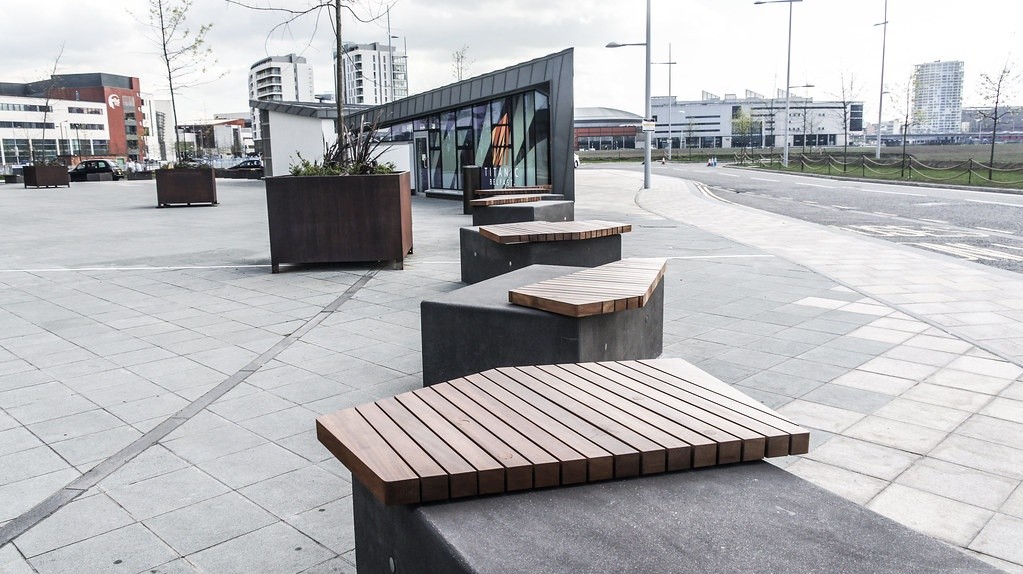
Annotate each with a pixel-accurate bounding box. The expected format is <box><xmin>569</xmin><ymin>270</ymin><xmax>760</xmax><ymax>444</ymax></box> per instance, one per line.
<box><xmin>127</xmin><ymin>158</ymin><xmax>135</xmax><ymax>173</ymax></box>
<box><xmin>134</xmin><ymin>161</ymin><xmax>142</xmax><ymax>172</ymax></box>
<box><xmin>604</xmin><ymin>145</ymin><xmax>608</xmax><ymax>150</ymax></box>
<box><xmin>708</xmin><ymin>157</ymin><xmax>717</xmax><ymax>166</ymax></box>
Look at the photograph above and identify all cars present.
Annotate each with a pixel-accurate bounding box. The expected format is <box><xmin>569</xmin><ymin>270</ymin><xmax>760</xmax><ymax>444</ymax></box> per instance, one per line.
<box><xmin>224</xmin><ymin>160</ymin><xmax>263</xmax><ymax>169</ymax></box>
<box><xmin>574</xmin><ymin>154</ymin><xmax>582</xmax><ymax>167</ymax></box>
<box><xmin>68</xmin><ymin>159</ymin><xmax>123</xmax><ymax>183</ymax></box>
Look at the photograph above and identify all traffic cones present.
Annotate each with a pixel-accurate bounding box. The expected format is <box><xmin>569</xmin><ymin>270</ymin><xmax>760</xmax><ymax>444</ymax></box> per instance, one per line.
<box><xmin>707</xmin><ymin>156</ymin><xmax>718</xmax><ymax>167</ymax></box>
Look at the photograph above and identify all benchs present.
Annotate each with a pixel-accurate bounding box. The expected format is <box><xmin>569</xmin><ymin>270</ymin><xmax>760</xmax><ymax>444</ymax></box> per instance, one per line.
<box><xmin>316</xmin><ymin>358</ymin><xmax>1007</xmax><ymax>573</ymax></box>
<box><xmin>420</xmin><ymin>258</ymin><xmax>669</xmax><ymax>388</ymax></box>
<box><xmin>470</xmin><ymin>187</ymin><xmax>574</xmax><ymax>226</ymax></box>
<box><xmin>460</xmin><ymin>218</ymin><xmax>632</xmax><ymax>285</ymax></box>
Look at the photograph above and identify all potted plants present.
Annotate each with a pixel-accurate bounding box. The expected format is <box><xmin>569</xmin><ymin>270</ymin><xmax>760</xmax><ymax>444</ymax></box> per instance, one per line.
<box><xmin>155</xmin><ymin>160</ymin><xmax>217</xmax><ymax>206</ymax></box>
<box><xmin>22</xmin><ymin>158</ymin><xmax>71</xmax><ymax>188</ymax></box>
<box><xmin>261</xmin><ymin>108</ymin><xmax>413</xmax><ymax>270</ymax></box>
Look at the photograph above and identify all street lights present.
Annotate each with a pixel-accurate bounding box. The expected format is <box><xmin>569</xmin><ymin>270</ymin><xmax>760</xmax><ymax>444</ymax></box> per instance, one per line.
<box><xmin>388</xmin><ymin>35</ymin><xmax>399</xmax><ymax>101</ymax></box>
<box><xmin>199</xmin><ymin>130</ymin><xmax>203</xmax><ymax>155</ymax></box>
<box><xmin>753</xmin><ymin>0</ymin><xmax>815</xmax><ymax>166</ymax></box>
<box><xmin>651</xmin><ymin>62</ymin><xmax>671</xmax><ymax>161</ymax></box>
<box><xmin>56</xmin><ymin>125</ymin><xmax>70</xmax><ymax>156</ymax></box>
<box><xmin>873</xmin><ymin>21</ymin><xmax>888</xmax><ymax>158</ymax></box>
<box><xmin>977</xmin><ymin>120</ymin><xmax>982</xmax><ymax>140</ymax></box>
<box><xmin>606</xmin><ymin>40</ymin><xmax>650</xmax><ymax>190</ymax></box>
<box><xmin>181</xmin><ymin>126</ymin><xmax>190</xmax><ymax>160</ymax></box>
<box><xmin>60</xmin><ymin>120</ymin><xmax>69</xmax><ymax>166</ymax></box>
<box><xmin>12</xmin><ymin>126</ymin><xmax>21</xmax><ymax>165</ymax></box>
